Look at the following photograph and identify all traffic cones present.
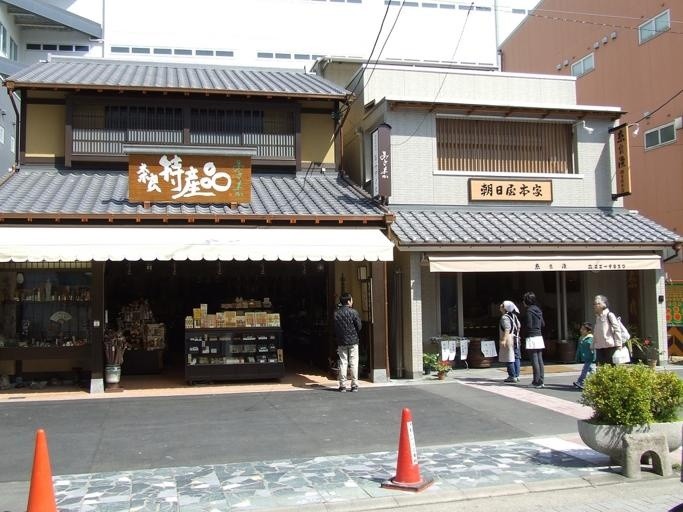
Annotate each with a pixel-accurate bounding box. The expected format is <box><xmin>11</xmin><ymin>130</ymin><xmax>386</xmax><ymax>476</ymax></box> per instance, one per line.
<box><xmin>381</xmin><ymin>408</ymin><xmax>434</xmax><ymax>492</ymax></box>
<box><xmin>25</xmin><ymin>428</ymin><xmax>58</xmax><ymax>512</ymax></box>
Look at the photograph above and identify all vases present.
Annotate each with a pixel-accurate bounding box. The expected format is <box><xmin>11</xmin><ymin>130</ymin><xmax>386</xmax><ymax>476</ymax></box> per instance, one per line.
<box><xmin>647</xmin><ymin>359</ymin><xmax>656</xmax><ymax>369</ymax></box>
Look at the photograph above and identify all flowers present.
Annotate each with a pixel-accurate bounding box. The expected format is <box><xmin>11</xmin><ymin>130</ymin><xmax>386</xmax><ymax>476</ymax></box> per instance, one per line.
<box><xmin>625</xmin><ymin>334</ymin><xmax>664</xmax><ymax>360</ymax></box>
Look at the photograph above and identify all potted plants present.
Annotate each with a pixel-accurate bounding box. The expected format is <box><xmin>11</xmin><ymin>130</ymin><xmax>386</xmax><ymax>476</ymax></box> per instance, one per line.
<box><xmin>423</xmin><ymin>352</ymin><xmax>452</xmax><ymax>381</ymax></box>
<box><xmin>577</xmin><ymin>361</ymin><xmax>682</xmax><ymax>466</ymax></box>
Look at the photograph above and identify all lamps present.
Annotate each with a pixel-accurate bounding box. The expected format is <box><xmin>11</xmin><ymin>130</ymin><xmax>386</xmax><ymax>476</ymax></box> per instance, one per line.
<box><xmin>572</xmin><ymin>120</ymin><xmax>594</xmax><ymax>135</ymax></box>
<box><xmin>628</xmin><ymin>122</ymin><xmax>640</xmax><ymax>138</ymax></box>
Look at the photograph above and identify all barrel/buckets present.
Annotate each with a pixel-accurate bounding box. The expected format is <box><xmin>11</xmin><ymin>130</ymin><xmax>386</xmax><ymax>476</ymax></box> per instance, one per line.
<box><xmin>105</xmin><ymin>364</ymin><xmax>121</xmax><ymax>383</ymax></box>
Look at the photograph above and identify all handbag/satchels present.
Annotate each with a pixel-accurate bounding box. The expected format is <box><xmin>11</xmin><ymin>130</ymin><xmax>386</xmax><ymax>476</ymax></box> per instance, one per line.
<box><xmin>607</xmin><ymin>311</ymin><xmax>630</xmax><ymax>344</ymax></box>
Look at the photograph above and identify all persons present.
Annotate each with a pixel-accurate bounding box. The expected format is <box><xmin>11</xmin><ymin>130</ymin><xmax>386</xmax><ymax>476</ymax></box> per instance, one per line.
<box><xmin>572</xmin><ymin>322</ymin><xmax>596</xmax><ymax>390</ymax></box>
<box><xmin>331</xmin><ymin>292</ymin><xmax>362</xmax><ymax>393</ymax></box>
<box><xmin>591</xmin><ymin>293</ymin><xmax>623</xmax><ymax>367</ymax></box>
<box><xmin>521</xmin><ymin>291</ymin><xmax>545</xmax><ymax>388</ymax></box>
<box><xmin>498</xmin><ymin>299</ymin><xmax>522</xmax><ymax>383</ymax></box>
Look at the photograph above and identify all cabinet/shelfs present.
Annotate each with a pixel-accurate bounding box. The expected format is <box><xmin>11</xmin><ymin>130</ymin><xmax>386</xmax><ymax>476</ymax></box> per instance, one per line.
<box><xmin>183</xmin><ymin>326</ymin><xmax>286</xmax><ymax>386</ymax></box>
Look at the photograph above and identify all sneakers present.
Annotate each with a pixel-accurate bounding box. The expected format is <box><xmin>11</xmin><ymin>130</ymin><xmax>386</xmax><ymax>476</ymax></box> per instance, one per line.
<box><xmin>572</xmin><ymin>381</ymin><xmax>584</xmax><ymax>389</ymax></box>
<box><xmin>338</xmin><ymin>385</ymin><xmax>358</xmax><ymax>392</ymax></box>
<box><xmin>527</xmin><ymin>381</ymin><xmax>544</xmax><ymax>388</ymax></box>
<box><xmin>503</xmin><ymin>376</ymin><xmax>520</xmax><ymax>384</ymax></box>
